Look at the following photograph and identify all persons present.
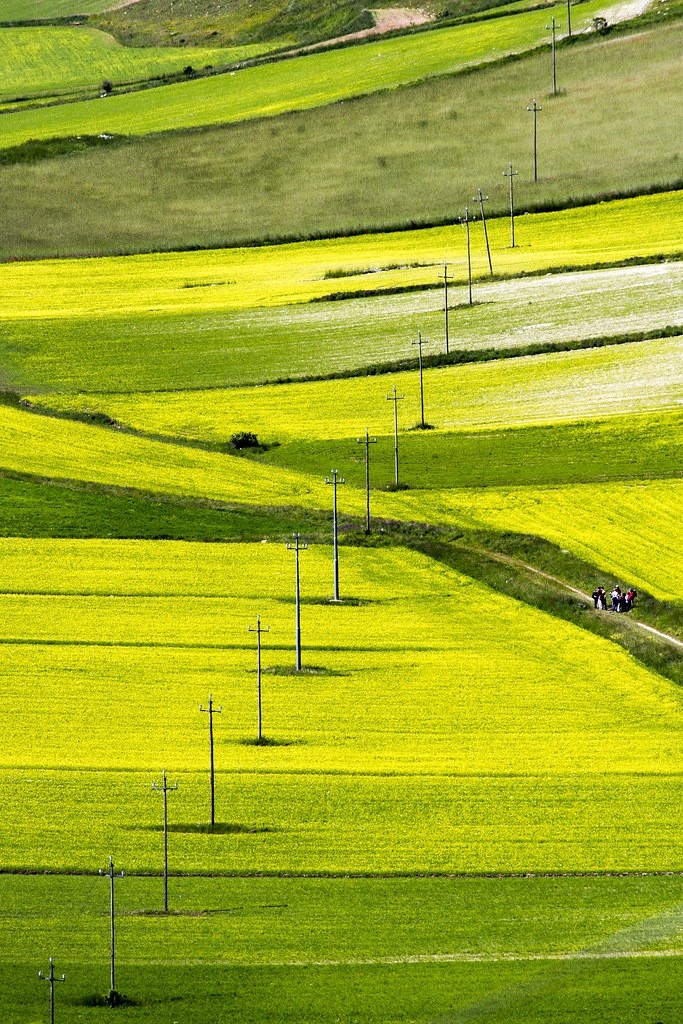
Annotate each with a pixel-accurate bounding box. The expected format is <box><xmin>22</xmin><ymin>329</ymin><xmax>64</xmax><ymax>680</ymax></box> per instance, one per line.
<box><xmin>592</xmin><ymin>587</ymin><xmax>607</xmax><ymax>610</ymax></box>
<box><xmin>609</xmin><ymin>585</ymin><xmax>638</xmax><ymax>612</ymax></box>
